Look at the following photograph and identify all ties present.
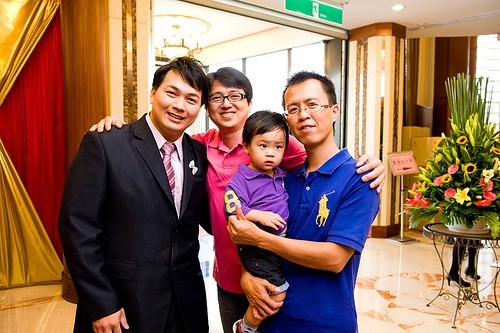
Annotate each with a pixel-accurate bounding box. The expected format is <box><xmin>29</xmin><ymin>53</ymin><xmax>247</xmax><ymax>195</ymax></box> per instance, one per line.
<box><xmin>160</xmin><ymin>142</ymin><xmax>176</xmax><ymax>199</ymax></box>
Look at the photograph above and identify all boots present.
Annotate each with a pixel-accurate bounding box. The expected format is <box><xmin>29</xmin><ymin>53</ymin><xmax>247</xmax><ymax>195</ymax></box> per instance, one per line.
<box><xmin>447</xmin><ymin>243</ymin><xmax>470</xmax><ymax>287</ymax></box>
<box><xmin>464</xmin><ymin>245</ymin><xmax>480</xmax><ymax>280</ymax></box>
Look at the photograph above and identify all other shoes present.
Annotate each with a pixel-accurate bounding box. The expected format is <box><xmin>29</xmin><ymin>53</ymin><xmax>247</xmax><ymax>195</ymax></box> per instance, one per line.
<box><xmin>232</xmin><ymin>319</ymin><xmax>259</xmax><ymax>333</ymax></box>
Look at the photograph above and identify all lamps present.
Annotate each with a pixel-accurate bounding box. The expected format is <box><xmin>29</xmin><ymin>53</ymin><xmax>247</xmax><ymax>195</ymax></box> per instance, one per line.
<box><xmin>154</xmin><ymin>13</ymin><xmax>211</xmax><ymax>66</ymax></box>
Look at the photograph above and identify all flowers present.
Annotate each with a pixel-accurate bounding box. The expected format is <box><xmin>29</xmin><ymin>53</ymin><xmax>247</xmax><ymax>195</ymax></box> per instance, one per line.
<box><xmin>393</xmin><ymin>72</ymin><xmax>500</xmax><ymax>238</ymax></box>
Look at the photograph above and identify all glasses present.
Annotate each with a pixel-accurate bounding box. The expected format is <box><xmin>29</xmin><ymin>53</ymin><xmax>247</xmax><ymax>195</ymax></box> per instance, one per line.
<box><xmin>208</xmin><ymin>92</ymin><xmax>247</xmax><ymax>103</ymax></box>
<box><xmin>284</xmin><ymin>103</ymin><xmax>334</xmax><ymax>117</ymax></box>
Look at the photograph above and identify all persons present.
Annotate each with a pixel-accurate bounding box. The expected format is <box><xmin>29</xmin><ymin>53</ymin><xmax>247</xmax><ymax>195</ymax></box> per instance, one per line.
<box><xmin>57</xmin><ymin>56</ymin><xmax>214</xmax><ymax>333</ymax></box>
<box><xmin>447</xmin><ymin>238</ymin><xmax>481</xmax><ymax>288</ymax></box>
<box><xmin>89</xmin><ymin>67</ymin><xmax>386</xmax><ymax>333</ymax></box>
<box><xmin>227</xmin><ymin>70</ymin><xmax>380</xmax><ymax>333</ymax></box>
<box><xmin>225</xmin><ymin>109</ymin><xmax>289</xmax><ymax>333</ymax></box>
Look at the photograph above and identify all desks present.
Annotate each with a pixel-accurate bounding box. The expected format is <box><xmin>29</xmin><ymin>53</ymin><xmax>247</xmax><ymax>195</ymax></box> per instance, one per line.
<box><xmin>423</xmin><ymin>222</ymin><xmax>500</xmax><ymax>328</ymax></box>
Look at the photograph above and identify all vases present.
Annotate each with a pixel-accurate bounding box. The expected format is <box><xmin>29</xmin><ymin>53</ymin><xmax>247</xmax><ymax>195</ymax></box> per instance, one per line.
<box><xmin>444</xmin><ymin>215</ymin><xmax>491</xmax><ymax>233</ymax></box>
